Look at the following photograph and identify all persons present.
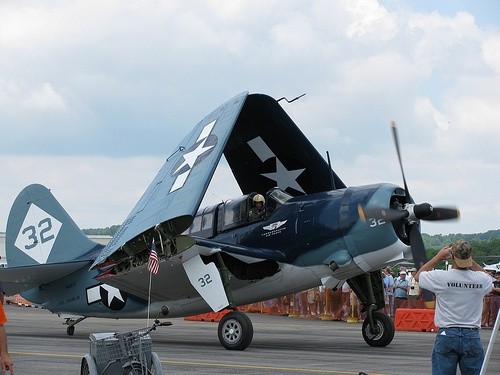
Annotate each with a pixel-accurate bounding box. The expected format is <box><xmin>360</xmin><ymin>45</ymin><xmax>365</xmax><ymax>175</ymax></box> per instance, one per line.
<box><xmin>0</xmin><ymin>295</ymin><xmax>13</xmax><ymax>375</ymax></box>
<box><xmin>237</xmin><ymin>266</ymin><xmax>500</xmax><ymax>327</ymax></box>
<box><xmin>248</xmin><ymin>194</ymin><xmax>266</xmax><ymax>219</ymax></box>
<box><xmin>415</xmin><ymin>239</ymin><xmax>493</xmax><ymax>375</ymax></box>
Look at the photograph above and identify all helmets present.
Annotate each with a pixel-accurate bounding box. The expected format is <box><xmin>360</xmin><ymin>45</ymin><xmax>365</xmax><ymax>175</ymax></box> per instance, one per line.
<box><xmin>252</xmin><ymin>194</ymin><xmax>265</xmax><ymax>206</ymax></box>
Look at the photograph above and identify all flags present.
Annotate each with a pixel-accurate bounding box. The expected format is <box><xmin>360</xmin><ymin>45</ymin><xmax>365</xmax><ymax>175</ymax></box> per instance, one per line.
<box><xmin>147</xmin><ymin>240</ymin><xmax>160</xmax><ymax>275</ymax></box>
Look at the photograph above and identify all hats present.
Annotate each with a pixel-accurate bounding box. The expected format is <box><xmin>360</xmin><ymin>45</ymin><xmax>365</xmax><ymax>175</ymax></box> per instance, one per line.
<box><xmin>452</xmin><ymin>239</ymin><xmax>473</xmax><ymax>268</ymax></box>
<box><xmin>408</xmin><ymin>268</ymin><xmax>417</xmax><ymax>272</ymax></box>
<box><xmin>400</xmin><ymin>271</ymin><xmax>406</xmax><ymax>275</ymax></box>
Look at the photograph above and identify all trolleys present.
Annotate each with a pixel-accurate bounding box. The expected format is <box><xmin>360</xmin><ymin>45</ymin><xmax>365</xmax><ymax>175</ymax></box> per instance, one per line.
<box><xmin>80</xmin><ymin>318</ymin><xmax>173</xmax><ymax>375</ymax></box>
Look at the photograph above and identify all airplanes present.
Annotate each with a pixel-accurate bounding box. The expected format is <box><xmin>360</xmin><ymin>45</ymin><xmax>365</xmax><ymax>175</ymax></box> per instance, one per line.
<box><xmin>1</xmin><ymin>91</ymin><xmax>460</xmax><ymax>350</ymax></box>
